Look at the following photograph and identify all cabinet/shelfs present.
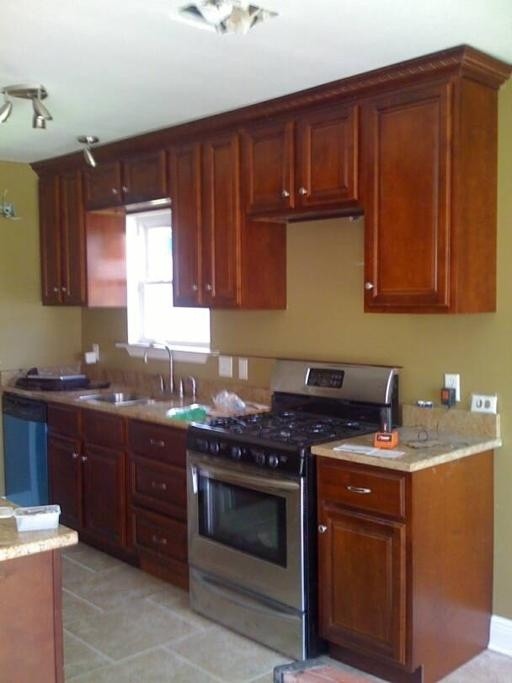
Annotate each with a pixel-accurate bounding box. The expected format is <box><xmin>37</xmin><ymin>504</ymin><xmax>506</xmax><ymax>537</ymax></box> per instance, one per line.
<box><xmin>0</xmin><ymin>547</ymin><xmax>64</xmax><ymax>683</ymax></box>
<box><xmin>45</xmin><ymin>400</ymin><xmax>189</xmax><ymax>591</ymax></box>
<box><xmin>317</xmin><ymin>450</ymin><xmax>494</xmax><ymax>683</ymax></box>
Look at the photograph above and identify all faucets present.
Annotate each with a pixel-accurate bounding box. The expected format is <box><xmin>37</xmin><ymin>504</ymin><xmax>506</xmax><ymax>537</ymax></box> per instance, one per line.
<box><xmin>144</xmin><ymin>341</ymin><xmax>174</xmax><ymax>394</ymax></box>
<box><xmin>186</xmin><ymin>375</ymin><xmax>198</xmax><ymax>402</ymax></box>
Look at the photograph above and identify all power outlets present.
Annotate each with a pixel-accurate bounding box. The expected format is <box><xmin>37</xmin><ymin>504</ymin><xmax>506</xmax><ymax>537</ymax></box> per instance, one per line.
<box><xmin>445</xmin><ymin>374</ymin><xmax>461</xmax><ymax>401</ymax></box>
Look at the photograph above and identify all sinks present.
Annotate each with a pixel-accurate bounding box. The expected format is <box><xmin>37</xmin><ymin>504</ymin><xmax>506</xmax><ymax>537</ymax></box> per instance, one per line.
<box><xmin>75</xmin><ymin>389</ymin><xmax>152</xmax><ymax>406</ymax></box>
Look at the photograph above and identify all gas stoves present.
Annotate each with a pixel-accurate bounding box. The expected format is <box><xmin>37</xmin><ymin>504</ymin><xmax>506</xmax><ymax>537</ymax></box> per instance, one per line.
<box><xmin>188</xmin><ymin>408</ymin><xmax>379</xmax><ymax>477</ymax></box>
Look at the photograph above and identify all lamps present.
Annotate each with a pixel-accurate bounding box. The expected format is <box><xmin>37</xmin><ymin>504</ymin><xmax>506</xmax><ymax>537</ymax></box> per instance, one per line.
<box><xmin>0</xmin><ymin>83</ymin><xmax>52</xmax><ymax>129</ymax></box>
<box><xmin>78</xmin><ymin>135</ymin><xmax>100</xmax><ymax>167</ymax></box>
<box><xmin>173</xmin><ymin>0</ymin><xmax>279</xmax><ymax>36</ymax></box>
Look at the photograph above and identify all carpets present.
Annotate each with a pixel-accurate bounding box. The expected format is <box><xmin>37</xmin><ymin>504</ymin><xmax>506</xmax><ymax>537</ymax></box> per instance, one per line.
<box><xmin>273</xmin><ymin>649</ymin><xmax>511</xmax><ymax>683</ymax></box>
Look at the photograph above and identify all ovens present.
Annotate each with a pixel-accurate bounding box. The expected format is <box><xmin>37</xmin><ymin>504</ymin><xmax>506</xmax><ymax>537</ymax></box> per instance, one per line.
<box><xmin>183</xmin><ymin>447</ymin><xmax>328</xmax><ymax>662</ymax></box>
